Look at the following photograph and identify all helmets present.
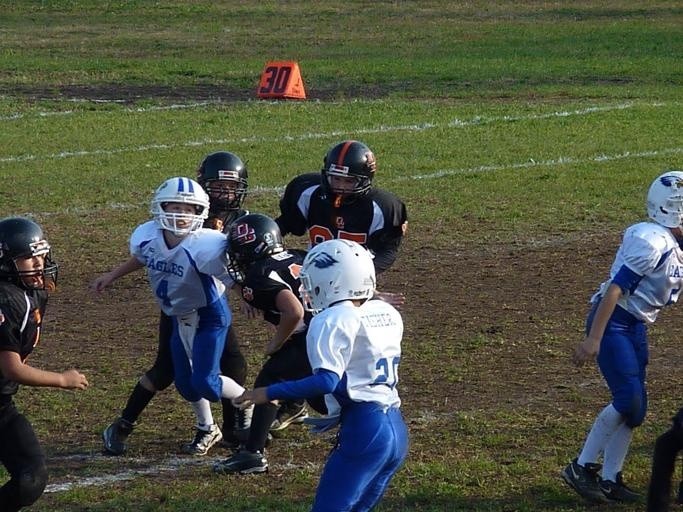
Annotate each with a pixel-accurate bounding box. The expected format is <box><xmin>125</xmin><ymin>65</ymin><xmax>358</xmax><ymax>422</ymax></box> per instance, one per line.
<box><xmin>151</xmin><ymin>141</ymin><xmax>375</xmax><ymax>312</ymax></box>
<box><xmin>646</xmin><ymin>171</ymin><xmax>682</xmax><ymax>228</ymax></box>
<box><xmin>0</xmin><ymin>218</ymin><xmax>58</xmax><ymax>290</ymax></box>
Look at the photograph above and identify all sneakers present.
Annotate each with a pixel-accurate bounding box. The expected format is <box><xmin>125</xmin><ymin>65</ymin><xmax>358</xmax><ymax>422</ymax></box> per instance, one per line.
<box><xmin>563</xmin><ymin>457</ymin><xmax>643</xmax><ymax>506</ymax></box>
<box><xmin>105</xmin><ymin>418</ymin><xmax>132</xmax><ymax>453</ymax></box>
<box><xmin>183</xmin><ymin>394</ymin><xmax>309</xmax><ymax>474</ymax></box>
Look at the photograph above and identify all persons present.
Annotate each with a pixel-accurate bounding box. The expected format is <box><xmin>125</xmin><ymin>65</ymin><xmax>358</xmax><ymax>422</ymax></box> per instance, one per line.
<box><xmin>0</xmin><ymin>216</ymin><xmax>91</xmax><ymax>510</ymax></box>
<box><xmin>561</xmin><ymin>168</ymin><xmax>680</xmax><ymax>504</ymax></box>
<box><xmin>268</xmin><ymin>138</ymin><xmax>410</xmax><ymax>433</ymax></box>
<box><xmin>235</xmin><ymin>239</ymin><xmax>414</xmax><ymax>512</ymax></box>
<box><xmin>213</xmin><ymin>212</ymin><xmax>406</xmax><ymax>475</ymax></box>
